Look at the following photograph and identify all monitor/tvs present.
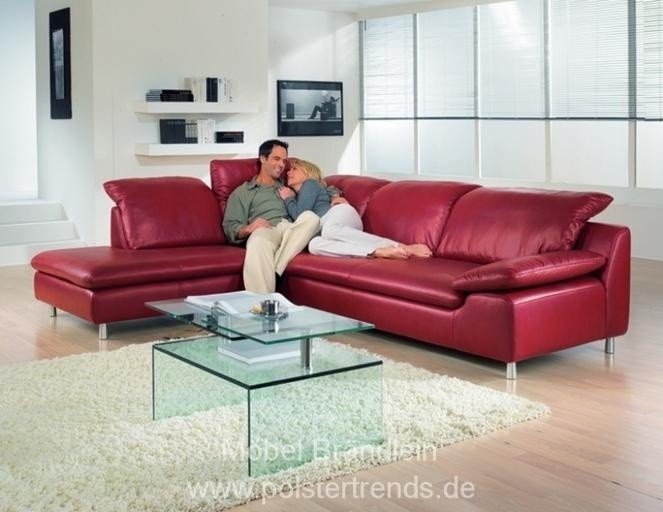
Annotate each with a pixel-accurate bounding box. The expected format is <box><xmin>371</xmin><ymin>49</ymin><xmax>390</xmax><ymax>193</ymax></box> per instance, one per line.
<box><xmin>277</xmin><ymin>79</ymin><xmax>343</xmax><ymax>136</ymax></box>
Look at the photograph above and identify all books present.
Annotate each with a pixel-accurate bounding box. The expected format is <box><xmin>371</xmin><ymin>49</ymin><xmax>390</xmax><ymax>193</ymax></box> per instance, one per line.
<box><xmin>218</xmin><ymin>337</ymin><xmax>316</xmax><ymax>364</ymax></box>
<box><xmin>146</xmin><ymin>77</ymin><xmax>232</xmax><ymax>143</ymax></box>
<box><xmin>187</xmin><ymin>290</ymin><xmax>299</xmax><ymax>318</ymax></box>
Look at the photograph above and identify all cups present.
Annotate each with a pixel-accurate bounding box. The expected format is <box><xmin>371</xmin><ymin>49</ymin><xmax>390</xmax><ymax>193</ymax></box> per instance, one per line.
<box><xmin>262</xmin><ymin>321</ymin><xmax>277</xmax><ymax>334</ymax></box>
<box><xmin>263</xmin><ymin>300</ymin><xmax>279</xmax><ymax>313</ymax></box>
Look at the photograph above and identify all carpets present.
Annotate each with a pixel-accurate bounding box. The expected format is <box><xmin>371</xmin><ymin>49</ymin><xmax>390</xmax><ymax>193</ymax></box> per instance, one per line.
<box><xmin>0</xmin><ymin>333</ymin><xmax>551</xmax><ymax>511</ymax></box>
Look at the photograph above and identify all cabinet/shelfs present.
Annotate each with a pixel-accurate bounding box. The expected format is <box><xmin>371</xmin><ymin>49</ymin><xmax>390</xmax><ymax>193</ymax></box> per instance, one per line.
<box><xmin>130</xmin><ymin>101</ymin><xmax>257</xmax><ymax>157</ymax></box>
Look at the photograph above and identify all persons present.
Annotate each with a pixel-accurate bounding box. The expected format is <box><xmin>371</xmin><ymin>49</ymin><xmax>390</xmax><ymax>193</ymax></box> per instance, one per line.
<box><xmin>277</xmin><ymin>159</ymin><xmax>433</xmax><ymax>260</ymax></box>
<box><xmin>308</xmin><ymin>96</ymin><xmax>335</xmax><ymax>119</ymax></box>
<box><xmin>221</xmin><ymin>140</ymin><xmax>349</xmax><ymax>295</ymax></box>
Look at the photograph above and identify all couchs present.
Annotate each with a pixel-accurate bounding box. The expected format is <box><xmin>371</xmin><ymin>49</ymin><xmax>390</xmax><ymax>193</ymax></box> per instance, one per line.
<box><xmin>31</xmin><ymin>152</ymin><xmax>632</xmax><ymax>383</ymax></box>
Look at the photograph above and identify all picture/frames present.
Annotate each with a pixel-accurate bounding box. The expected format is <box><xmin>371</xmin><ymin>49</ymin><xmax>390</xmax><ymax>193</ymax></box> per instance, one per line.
<box><xmin>47</xmin><ymin>6</ymin><xmax>74</xmax><ymax>121</ymax></box>
